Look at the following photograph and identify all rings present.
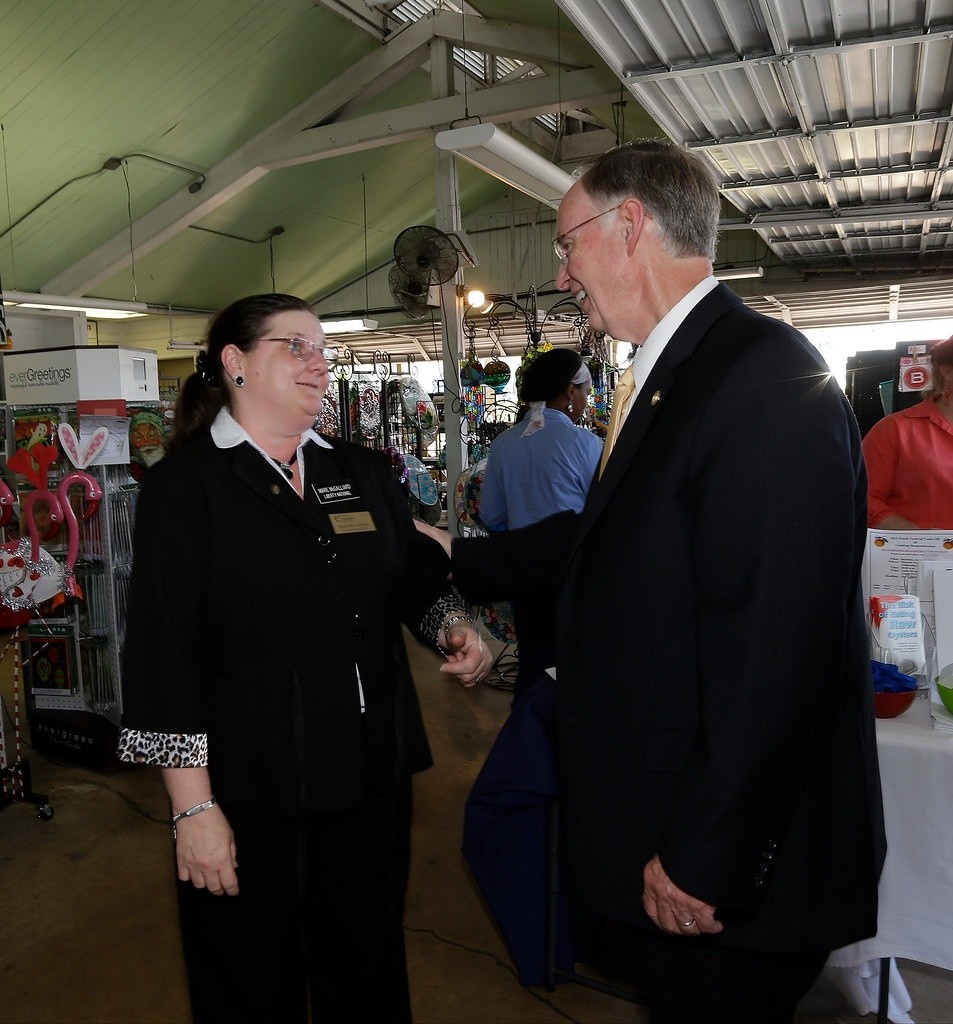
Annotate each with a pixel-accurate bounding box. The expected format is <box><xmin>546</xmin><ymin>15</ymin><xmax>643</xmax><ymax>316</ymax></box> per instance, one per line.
<box><xmin>677</xmin><ymin>918</ymin><xmax>695</xmax><ymax>927</ymax></box>
<box><xmin>476</xmin><ymin>676</ymin><xmax>480</xmax><ymax>682</ymax></box>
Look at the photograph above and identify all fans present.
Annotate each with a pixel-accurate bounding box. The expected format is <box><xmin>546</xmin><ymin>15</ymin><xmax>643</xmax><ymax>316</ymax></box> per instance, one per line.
<box><xmin>393</xmin><ymin>225</ymin><xmax>459</xmax><ymax>286</ymax></box>
<box><xmin>388</xmin><ymin>265</ymin><xmax>432</xmax><ymax>320</ymax></box>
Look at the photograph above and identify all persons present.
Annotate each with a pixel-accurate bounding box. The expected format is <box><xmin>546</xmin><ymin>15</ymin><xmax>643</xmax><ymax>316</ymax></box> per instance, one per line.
<box><xmin>862</xmin><ymin>335</ymin><xmax>953</xmax><ymax>531</ymax></box>
<box><xmin>118</xmin><ymin>292</ymin><xmax>494</xmax><ymax>1024</ymax></box>
<box><xmin>479</xmin><ymin>348</ymin><xmax>604</xmax><ymax>712</ymax></box>
<box><xmin>410</xmin><ymin>135</ymin><xmax>890</xmax><ymax>1024</ymax></box>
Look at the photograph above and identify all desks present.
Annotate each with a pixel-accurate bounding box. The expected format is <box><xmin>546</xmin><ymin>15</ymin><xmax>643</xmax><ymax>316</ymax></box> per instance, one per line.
<box><xmin>869</xmin><ymin>696</ymin><xmax>953</xmax><ymax>1022</ymax></box>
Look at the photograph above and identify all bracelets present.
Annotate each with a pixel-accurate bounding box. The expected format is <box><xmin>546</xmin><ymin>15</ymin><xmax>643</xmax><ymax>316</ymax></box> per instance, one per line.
<box><xmin>444</xmin><ymin>616</ymin><xmax>477</xmax><ymax>647</ymax></box>
<box><xmin>169</xmin><ymin>794</ymin><xmax>218</xmax><ymax>839</ymax></box>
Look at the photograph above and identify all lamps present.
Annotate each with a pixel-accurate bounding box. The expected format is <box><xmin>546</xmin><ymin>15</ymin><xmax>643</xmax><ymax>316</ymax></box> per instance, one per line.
<box><xmin>456</xmin><ymin>284</ymin><xmax>485</xmax><ymax>309</ymax></box>
<box><xmin>435</xmin><ymin>115</ymin><xmax>578</xmax><ymax>212</ymax></box>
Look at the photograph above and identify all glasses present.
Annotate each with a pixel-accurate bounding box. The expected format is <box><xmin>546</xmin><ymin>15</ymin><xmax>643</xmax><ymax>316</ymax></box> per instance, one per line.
<box><xmin>252</xmin><ymin>336</ymin><xmax>339</xmax><ymax>372</ymax></box>
<box><xmin>551</xmin><ymin>203</ymin><xmax>652</xmax><ymax>264</ymax></box>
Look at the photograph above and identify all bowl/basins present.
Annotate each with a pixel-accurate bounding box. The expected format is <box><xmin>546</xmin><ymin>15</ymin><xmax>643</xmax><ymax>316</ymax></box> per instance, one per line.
<box><xmin>934</xmin><ymin>675</ymin><xmax>953</xmax><ymax>715</ymax></box>
<box><xmin>871</xmin><ymin>690</ymin><xmax>918</xmax><ymax>721</ymax></box>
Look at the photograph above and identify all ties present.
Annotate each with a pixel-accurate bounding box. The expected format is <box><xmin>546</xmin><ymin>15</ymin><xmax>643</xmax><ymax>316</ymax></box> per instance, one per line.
<box><xmin>598</xmin><ymin>361</ymin><xmax>634</xmax><ymax>482</ymax></box>
